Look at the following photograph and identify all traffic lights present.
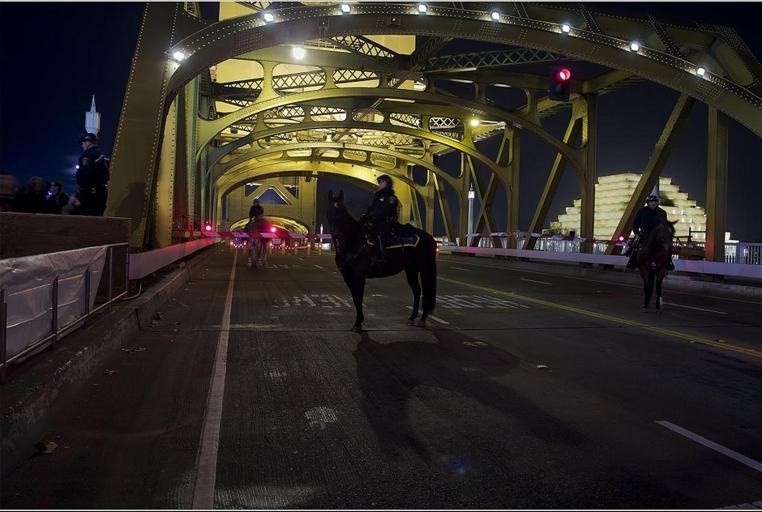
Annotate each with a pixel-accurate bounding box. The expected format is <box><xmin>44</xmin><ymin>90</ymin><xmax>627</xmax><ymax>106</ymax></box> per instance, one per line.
<box><xmin>547</xmin><ymin>65</ymin><xmax>580</xmax><ymax>102</ymax></box>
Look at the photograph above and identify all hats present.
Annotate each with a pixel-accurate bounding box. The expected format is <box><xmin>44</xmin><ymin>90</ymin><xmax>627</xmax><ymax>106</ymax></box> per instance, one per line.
<box><xmin>252</xmin><ymin>198</ymin><xmax>260</xmax><ymax>204</ymax></box>
<box><xmin>377</xmin><ymin>174</ymin><xmax>393</xmax><ymax>185</ymax></box>
<box><xmin>646</xmin><ymin>194</ymin><xmax>658</xmax><ymax>202</ymax></box>
<box><xmin>78</xmin><ymin>133</ymin><xmax>97</xmax><ymax>146</ymax></box>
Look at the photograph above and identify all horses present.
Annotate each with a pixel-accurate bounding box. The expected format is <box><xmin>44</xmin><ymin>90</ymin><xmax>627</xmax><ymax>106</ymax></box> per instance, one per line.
<box><xmin>637</xmin><ymin>215</ymin><xmax>680</xmax><ymax>313</ymax></box>
<box><xmin>325</xmin><ymin>188</ymin><xmax>438</xmax><ymax>335</ymax></box>
<box><xmin>245</xmin><ymin>217</ymin><xmax>274</xmax><ymax>265</ymax></box>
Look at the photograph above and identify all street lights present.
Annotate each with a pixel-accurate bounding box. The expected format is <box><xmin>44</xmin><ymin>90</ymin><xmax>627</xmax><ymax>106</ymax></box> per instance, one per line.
<box><xmin>467</xmin><ymin>183</ymin><xmax>475</xmax><ymax>247</ymax></box>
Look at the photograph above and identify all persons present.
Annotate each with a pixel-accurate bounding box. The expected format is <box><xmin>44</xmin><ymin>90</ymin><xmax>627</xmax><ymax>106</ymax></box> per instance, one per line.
<box><xmin>631</xmin><ymin>195</ymin><xmax>667</xmax><ymax>240</ymax></box>
<box><xmin>39</xmin><ymin>180</ymin><xmax>70</xmax><ymax>214</ymax></box>
<box><xmin>359</xmin><ymin>175</ymin><xmax>399</xmax><ymax>258</ymax></box>
<box><xmin>247</xmin><ymin>197</ymin><xmax>265</xmax><ymax>222</ymax></box>
<box><xmin>62</xmin><ymin>133</ymin><xmax>111</xmax><ymax>215</ymax></box>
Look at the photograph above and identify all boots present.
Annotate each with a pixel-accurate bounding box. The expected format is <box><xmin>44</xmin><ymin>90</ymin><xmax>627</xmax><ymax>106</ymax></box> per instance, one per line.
<box><xmin>375</xmin><ymin>238</ymin><xmax>386</xmax><ymax>262</ymax></box>
